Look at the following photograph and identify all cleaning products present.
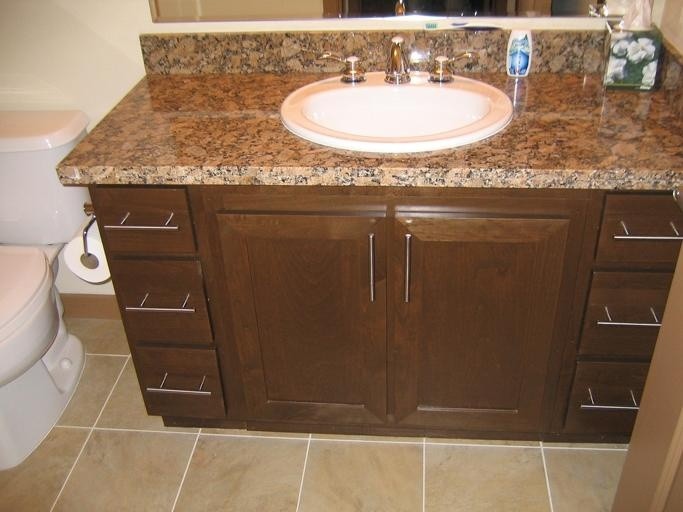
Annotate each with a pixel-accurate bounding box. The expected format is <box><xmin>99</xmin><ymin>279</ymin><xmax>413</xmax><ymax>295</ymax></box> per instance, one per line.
<box><xmin>507</xmin><ymin>30</ymin><xmax>533</xmax><ymax>77</ymax></box>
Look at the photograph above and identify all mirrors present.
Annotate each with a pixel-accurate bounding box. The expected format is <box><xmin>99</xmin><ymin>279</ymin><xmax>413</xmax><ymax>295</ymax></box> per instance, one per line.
<box><xmin>148</xmin><ymin>0</ymin><xmax>627</xmax><ymax>24</ymax></box>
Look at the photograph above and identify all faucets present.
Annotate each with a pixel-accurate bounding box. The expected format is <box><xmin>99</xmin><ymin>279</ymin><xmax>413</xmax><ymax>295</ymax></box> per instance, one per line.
<box><xmin>395</xmin><ymin>0</ymin><xmax>404</xmax><ymax>15</ymax></box>
<box><xmin>385</xmin><ymin>35</ymin><xmax>411</xmax><ymax>83</ymax></box>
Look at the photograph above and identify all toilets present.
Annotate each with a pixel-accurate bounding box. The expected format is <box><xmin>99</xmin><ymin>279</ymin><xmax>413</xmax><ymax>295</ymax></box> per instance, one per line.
<box><xmin>0</xmin><ymin>109</ymin><xmax>88</xmax><ymax>470</ymax></box>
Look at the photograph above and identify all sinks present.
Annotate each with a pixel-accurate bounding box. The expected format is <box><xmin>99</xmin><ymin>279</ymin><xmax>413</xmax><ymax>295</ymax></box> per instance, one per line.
<box><xmin>279</xmin><ymin>72</ymin><xmax>513</xmax><ymax>153</ymax></box>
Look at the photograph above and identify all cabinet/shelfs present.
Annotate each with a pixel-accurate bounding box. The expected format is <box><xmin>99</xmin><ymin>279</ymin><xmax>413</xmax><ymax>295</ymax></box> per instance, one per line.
<box><xmin>205</xmin><ymin>187</ymin><xmax>590</xmax><ymax>446</ymax></box>
<box><xmin>546</xmin><ymin>190</ymin><xmax>682</xmax><ymax>446</ymax></box>
<box><xmin>88</xmin><ymin>185</ymin><xmax>250</xmax><ymax>430</ymax></box>
